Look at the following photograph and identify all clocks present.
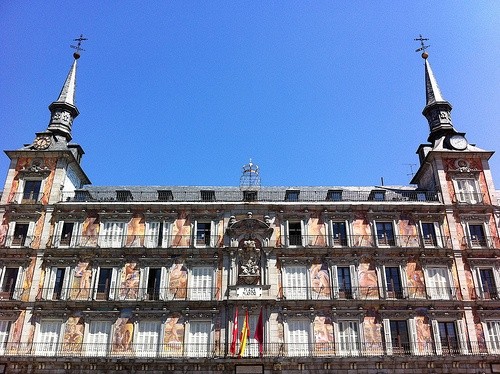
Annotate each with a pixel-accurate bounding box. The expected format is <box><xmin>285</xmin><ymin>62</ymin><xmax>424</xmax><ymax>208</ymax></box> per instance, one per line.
<box><xmin>33</xmin><ymin>135</ymin><xmax>51</xmax><ymax>149</ymax></box>
<box><xmin>449</xmin><ymin>135</ymin><xmax>468</xmax><ymax>150</ymax></box>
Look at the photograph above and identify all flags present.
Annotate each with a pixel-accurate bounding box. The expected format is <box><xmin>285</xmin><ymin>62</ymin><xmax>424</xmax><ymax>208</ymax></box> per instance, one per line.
<box><xmin>229</xmin><ymin>306</ymin><xmax>239</xmax><ymax>357</ymax></box>
<box><xmin>238</xmin><ymin>305</ymin><xmax>250</xmax><ymax>358</ymax></box>
<box><xmin>253</xmin><ymin>306</ymin><xmax>265</xmax><ymax>358</ymax></box>
